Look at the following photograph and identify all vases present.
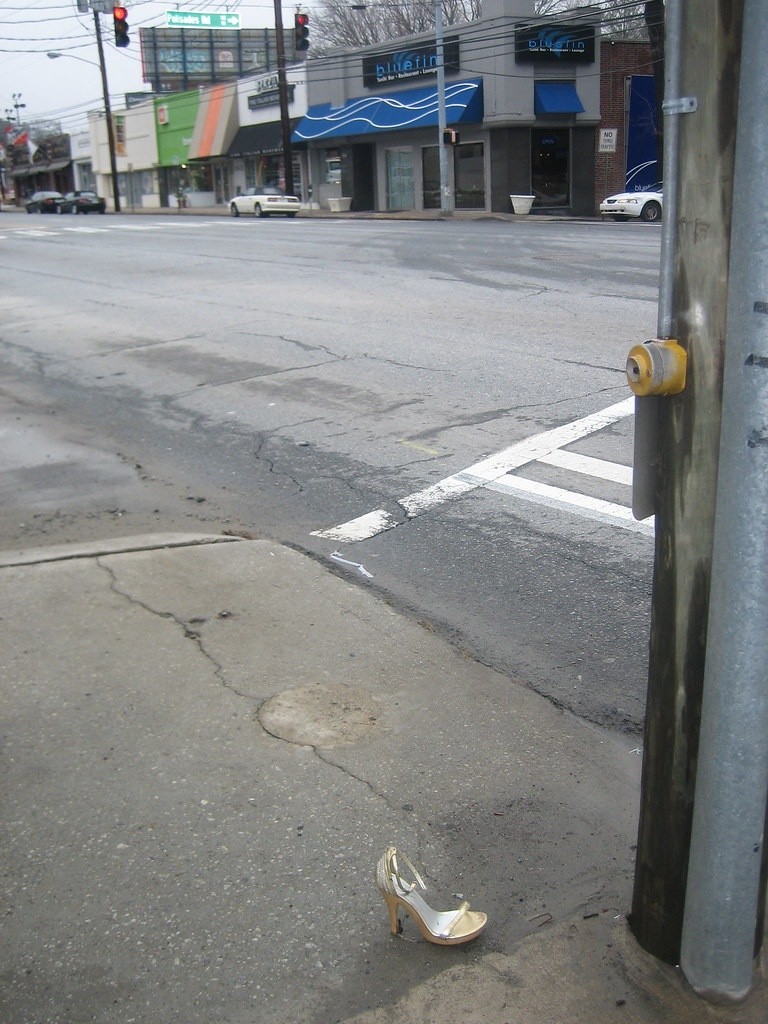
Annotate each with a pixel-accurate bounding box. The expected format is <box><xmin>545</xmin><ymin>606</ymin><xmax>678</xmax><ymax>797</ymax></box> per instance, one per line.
<box><xmin>327</xmin><ymin>197</ymin><xmax>352</xmax><ymax>212</ymax></box>
<box><xmin>178</xmin><ymin>200</ymin><xmax>186</xmax><ymax>208</ymax></box>
<box><xmin>511</xmin><ymin>194</ymin><xmax>535</xmax><ymax>216</ymax></box>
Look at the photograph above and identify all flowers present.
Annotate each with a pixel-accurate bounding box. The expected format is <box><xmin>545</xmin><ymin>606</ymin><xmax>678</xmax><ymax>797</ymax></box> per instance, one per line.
<box><xmin>176</xmin><ymin>189</ymin><xmax>187</xmax><ymax>200</ymax></box>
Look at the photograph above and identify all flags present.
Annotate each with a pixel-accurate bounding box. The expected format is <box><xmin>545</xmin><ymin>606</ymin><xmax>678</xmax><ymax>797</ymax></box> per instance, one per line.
<box><xmin>6</xmin><ymin>127</ymin><xmax>39</xmax><ymax>165</ymax></box>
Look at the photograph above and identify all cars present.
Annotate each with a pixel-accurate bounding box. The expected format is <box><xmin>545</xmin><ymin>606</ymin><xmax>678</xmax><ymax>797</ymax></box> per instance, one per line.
<box><xmin>62</xmin><ymin>190</ymin><xmax>106</xmax><ymax>215</ymax></box>
<box><xmin>599</xmin><ymin>180</ymin><xmax>664</xmax><ymax>222</ymax></box>
<box><xmin>25</xmin><ymin>190</ymin><xmax>72</xmax><ymax>215</ymax></box>
<box><xmin>227</xmin><ymin>185</ymin><xmax>302</xmax><ymax>219</ymax></box>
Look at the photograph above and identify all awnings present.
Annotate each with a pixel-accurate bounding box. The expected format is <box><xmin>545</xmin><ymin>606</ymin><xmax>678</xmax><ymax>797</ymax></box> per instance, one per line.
<box><xmin>14</xmin><ymin>161</ymin><xmax>72</xmax><ymax>178</ymax></box>
<box><xmin>533</xmin><ymin>82</ymin><xmax>586</xmax><ymax>114</ymax></box>
<box><xmin>290</xmin><ymin>75</ymin><xmax>485</xmax><ymax>144</ymax></box>
<box><xmin>226</xmin><ymin>114</ymin><xmax>308</xmax><ymax>158</ymax></box>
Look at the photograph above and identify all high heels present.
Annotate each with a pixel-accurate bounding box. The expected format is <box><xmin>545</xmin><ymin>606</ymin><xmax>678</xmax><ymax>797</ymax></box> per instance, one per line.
<box><xmin>376</xmin><ymin>847</ymin><xmax>488</xmax><ymax>946</ymax></box>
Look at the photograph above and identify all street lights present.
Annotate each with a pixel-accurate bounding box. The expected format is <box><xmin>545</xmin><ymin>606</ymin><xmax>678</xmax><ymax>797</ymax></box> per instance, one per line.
<box><xmin>47</xmin><ymin>51</ymin><xmax>121</xmax><ymax>213</ymax></box>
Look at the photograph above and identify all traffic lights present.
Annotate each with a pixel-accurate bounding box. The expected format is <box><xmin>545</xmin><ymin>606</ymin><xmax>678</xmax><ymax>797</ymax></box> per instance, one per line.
<box><xmin>113</xmin><ymin>6</ymin><xmax>131</xmax><ymax>48</ymax></box>
<box><xmin>294</xmin><ymin>14</ymin><xmax>309</xmax><ymax>52</ymax></box>
